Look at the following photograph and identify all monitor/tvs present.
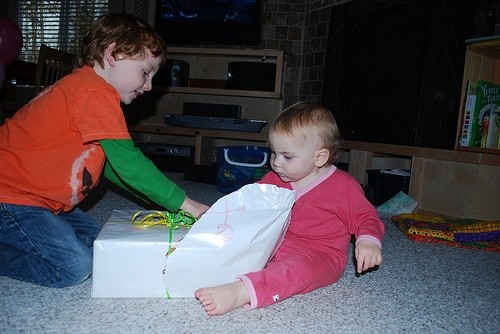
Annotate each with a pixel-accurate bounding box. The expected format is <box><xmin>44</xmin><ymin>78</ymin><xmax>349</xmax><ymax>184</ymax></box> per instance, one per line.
<box><xmin>155</xmin><ymin>0</ymin><xmax>264</xmax><ymax>47</ymax></box>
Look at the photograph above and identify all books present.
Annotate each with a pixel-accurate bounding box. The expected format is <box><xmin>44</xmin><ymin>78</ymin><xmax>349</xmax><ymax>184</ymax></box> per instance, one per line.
<box><xmin>460</xmin><ymin>80</ymin><xmax>500</xmax><ymax>149</ymax></box>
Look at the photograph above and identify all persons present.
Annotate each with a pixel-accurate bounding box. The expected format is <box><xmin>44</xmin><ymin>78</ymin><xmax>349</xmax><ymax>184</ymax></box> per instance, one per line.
<box><xmin>0</xmin><ymin>13</ymin><xmax>209</xmax><ymax>288</ymax></box>
<box><xmin>194</xmin><ymin>100</ymin><xmax>385</xmax><ymax>316</ymax></box>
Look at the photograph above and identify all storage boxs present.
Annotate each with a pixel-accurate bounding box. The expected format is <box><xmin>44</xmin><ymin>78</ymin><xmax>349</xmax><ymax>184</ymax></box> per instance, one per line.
<box><xmin>460</xmin><ymin>79</ymin><xmax>500</xmax><ymax>147</ymax></box>
<box><xmin>92</xmin><ymin>209</ymin><xmax>230</xmax><ymax>298</ymax></box>
<box><xmin>214</xmin><ymin>145</ymin><xmax>273</xmax><ymax>192</ymax></box>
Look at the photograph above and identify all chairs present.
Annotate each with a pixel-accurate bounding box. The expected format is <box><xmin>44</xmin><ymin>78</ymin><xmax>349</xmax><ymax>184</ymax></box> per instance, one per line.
<box><xmin>37</xmin><ymin>45</ymin><xmax>74</xmax><ymax>96</ymax></box>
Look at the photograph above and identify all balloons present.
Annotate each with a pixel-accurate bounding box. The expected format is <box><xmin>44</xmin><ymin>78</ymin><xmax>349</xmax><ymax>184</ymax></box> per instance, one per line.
<box><xmin>0</xmin><ymin>17</ymin><xmax>23</xmax><ymax>66</ymax></box>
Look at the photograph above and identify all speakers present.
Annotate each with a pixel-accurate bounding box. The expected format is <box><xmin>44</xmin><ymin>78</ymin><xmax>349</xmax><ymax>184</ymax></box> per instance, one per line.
<box><xmin>226</xmin><ymin>62</ymin><xmax>276</xmax><ymax>92</ymax></box>
<box><xmin>152</xmin><ymin>59</ymin><xmax>190</xmax><ymax>87</ymax></box>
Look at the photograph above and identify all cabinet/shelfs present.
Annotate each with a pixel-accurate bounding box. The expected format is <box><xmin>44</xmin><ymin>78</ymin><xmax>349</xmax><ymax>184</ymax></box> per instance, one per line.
<box><xmin>145</xmin><ymin>49</ymin><xmax>285</xmax><ymax>165</ymax></box>
<box><xmin>456</xmin><ymin>36</ymin><xmax>500</xmax><ymax>151</ymax></box>
